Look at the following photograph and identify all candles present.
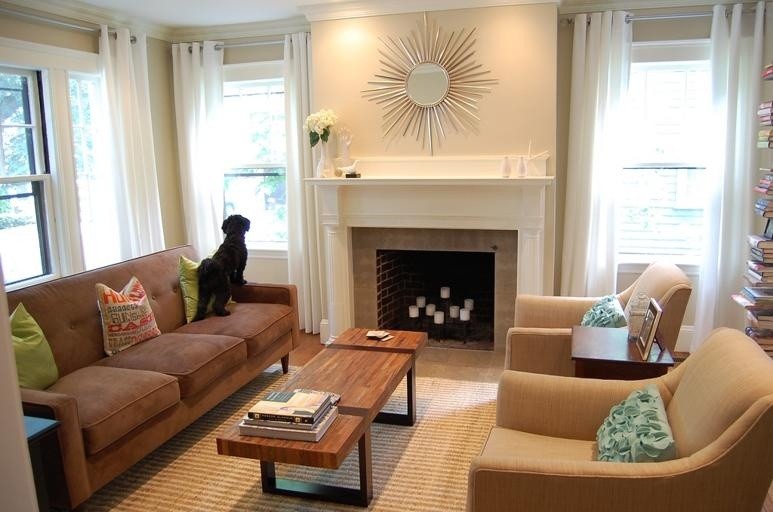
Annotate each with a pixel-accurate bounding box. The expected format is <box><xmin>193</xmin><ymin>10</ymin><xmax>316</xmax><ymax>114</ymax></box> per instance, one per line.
<box><xmin>406</xmin><ymin>283</ymin><xmax>476</xmax><ymax>328</ymax></box>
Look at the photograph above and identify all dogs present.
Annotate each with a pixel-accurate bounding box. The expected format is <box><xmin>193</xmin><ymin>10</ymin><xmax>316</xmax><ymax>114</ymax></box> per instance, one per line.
<box><xmin>188</xmin><ymin>214</ymin><xmax>252</xmax><ymax>323</ymax></box>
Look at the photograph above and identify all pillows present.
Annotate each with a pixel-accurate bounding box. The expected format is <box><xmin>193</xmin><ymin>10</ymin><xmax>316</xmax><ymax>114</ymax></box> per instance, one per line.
<box><xmin>176</xmin><ymin>252</ymin><xmax>235</xmax><ymax>326</ymax></box>
<box><xmin>7</xmin><ymin>301</ymin><xmax>61</xmax><ymax>394</ymax></box>
<box><xmin>580</xmin><ymin>291</ymin><xmax>629</xmax><ymax>329</ymax></box>
<box><xmin>593</xmin><ymin>380</ymin><xmax>681</xmax><ymax>468</ymax></box>
<box><xmin>91</xmin><ymin>274</ymin><xmax>163</xmax><ymax>362</ymax></box>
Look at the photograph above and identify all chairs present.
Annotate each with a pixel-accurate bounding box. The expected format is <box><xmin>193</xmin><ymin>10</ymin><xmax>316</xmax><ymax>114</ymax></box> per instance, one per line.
<box><xmin>503</xmin><ymin>252</ymin><xmax>693</xmax><ymax>380</ymax></box>
<box><xmin>462</xmin><ymin>325</ymin><xmax>773</xmax><ymax>512</ymax></box>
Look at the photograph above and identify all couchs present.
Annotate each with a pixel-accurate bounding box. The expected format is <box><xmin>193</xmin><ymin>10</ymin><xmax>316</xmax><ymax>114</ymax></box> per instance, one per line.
<box><xmin>6</xmin><ymin>242</ymin><xmax>302</xmax><ymax>511</ymax></box>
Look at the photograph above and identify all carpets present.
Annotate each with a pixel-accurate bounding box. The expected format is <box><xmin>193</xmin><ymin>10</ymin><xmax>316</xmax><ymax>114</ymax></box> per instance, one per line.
<box><xmin>66</xmin><ymin>359</ymin><xmax>504</xmax><ymax>510</ymax></box>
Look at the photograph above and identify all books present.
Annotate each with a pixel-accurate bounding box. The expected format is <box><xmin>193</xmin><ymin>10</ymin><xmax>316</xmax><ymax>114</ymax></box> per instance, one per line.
<box><xmin>729</xmin><ymin>60</ymin><xmax>771</xmax><ymax>360</ymax></box>
<box><xmin>237</xmin><ymin>390</ymin><xmax>338</xmax><ymax>444</ymax></box>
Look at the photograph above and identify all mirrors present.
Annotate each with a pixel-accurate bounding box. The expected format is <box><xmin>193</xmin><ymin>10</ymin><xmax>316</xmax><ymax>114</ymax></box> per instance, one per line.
<box><xmin>357</xmin><ymin>11</ymin><xmax>502</xmax><ymax>157</ymax></box>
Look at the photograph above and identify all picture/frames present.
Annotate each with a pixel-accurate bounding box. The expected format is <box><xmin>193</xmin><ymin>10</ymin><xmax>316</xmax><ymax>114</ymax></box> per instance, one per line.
<box><xmin>632</xmin><ymin>296</ymin><xmax>668</xmax><ymax>363</ymax></box>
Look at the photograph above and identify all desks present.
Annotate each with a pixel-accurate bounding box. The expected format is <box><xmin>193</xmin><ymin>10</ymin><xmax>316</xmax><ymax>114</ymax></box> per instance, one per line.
<box><xmin>568</xmin><ymin>324</ymin><xmax>676</xmax><ymax>378</ymax></box>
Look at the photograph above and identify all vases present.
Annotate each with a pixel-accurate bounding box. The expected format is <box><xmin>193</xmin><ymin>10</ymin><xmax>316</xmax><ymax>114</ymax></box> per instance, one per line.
<box><xmin>499</xmin><ymin>153</ymin><xmax>511</xmax><ymax>179</ymax></box>
<box><xmin>314</xmin><ymin>140</ymin><xmax>335</xmax><ymax>178</ymax></box>
<box><xmin>515</xmin><ymin>154</ymin><xmax>526</xmax><ymax>177</ymax></box>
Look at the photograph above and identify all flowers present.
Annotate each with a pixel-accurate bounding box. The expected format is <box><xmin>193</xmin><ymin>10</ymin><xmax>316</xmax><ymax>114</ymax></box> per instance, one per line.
<box><xmin>302</xmin><ymin>108</ymin><xmax>338</xmax><ymax>148</ymax></box>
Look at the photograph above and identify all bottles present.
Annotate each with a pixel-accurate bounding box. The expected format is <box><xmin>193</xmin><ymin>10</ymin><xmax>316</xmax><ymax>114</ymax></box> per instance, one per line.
<box><xmin>500</xmin><ymin>156</ymin><xmax>511</xmax><ymax>177</ymax></box>
<box><xmin>516</xmin><ymin>155</ymin><xmax>526</xmax><ymax>177</ymax></box>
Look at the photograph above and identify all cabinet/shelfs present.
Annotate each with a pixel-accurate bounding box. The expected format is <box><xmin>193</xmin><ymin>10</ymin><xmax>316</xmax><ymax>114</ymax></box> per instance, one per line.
<box><xmin>18</xmin><ymin>398</ymin><xmax>69</xmax><ymax>512</ymax></box>
